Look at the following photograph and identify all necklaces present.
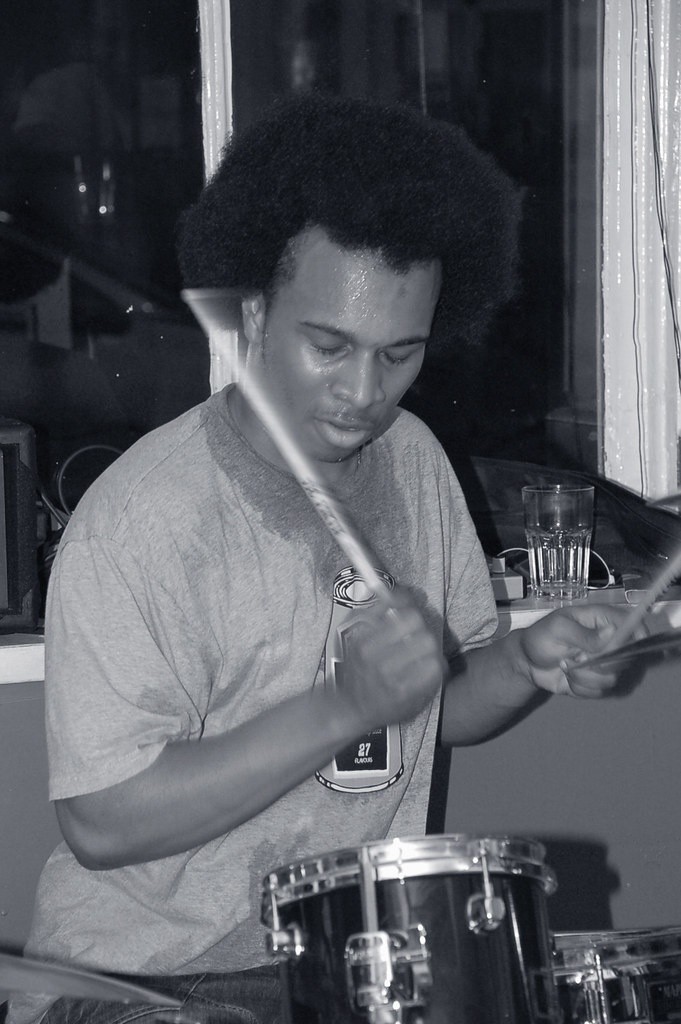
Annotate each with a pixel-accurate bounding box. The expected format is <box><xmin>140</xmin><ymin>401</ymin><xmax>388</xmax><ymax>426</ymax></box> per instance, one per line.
<box><xmin>356</xmin><ymin>449</ymin><xmax>360</xmax><ymax>464</ymax></box>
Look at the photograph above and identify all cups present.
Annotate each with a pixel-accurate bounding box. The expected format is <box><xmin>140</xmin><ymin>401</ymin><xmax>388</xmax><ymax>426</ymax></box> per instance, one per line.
<box><xmin>520</xmin><ymin>482</ymin><xmax>597</xmax><ymax>603</ymax></box>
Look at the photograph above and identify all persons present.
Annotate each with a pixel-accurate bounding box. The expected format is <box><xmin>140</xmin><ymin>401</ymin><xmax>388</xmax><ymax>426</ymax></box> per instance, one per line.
<box><xmin>0</xmin><ymin>94</ymin><xmax>648</xmax><ymax>1024</ymax></box>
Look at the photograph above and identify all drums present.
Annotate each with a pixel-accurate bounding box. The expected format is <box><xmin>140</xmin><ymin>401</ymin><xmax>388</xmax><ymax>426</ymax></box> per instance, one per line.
<box><xmin>0</xmin><ymin>948</ymin><xmax>209</xmax><ymax>1024</ymax></box>
<box><xmin>258</xmin><ymin>833</ymin><xmax>560</xmax><ymax>1023</ymax></box>
<box><xmin>540</xmin><ymin>919</ymin><xmax>678</xmax><ymax>1024</ymax></box>
